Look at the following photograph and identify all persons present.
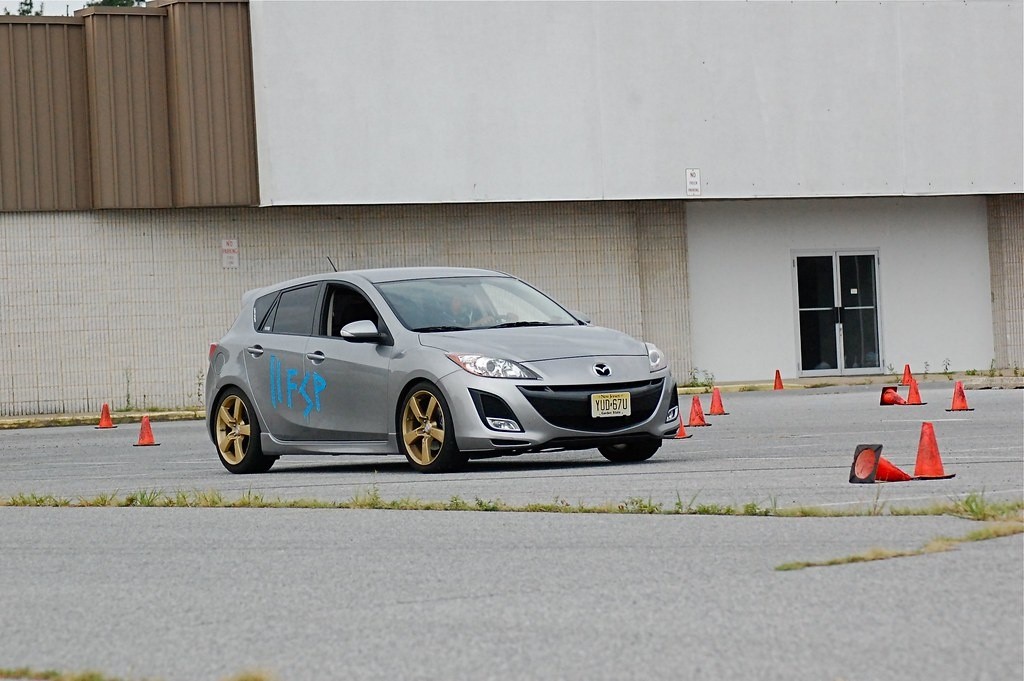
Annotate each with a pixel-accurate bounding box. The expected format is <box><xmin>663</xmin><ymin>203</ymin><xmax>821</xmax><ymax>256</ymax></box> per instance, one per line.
<box><xmin>435</xmin><ymin>283</ymin><xmax>518</xmax><ymax>327</ymax></box>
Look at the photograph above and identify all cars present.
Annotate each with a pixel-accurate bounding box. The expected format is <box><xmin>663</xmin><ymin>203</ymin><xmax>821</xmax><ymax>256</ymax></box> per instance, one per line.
<box><xmin>205</xmin><ymin>266</ymin><xmax>680</xmax><ymax>474</ymax></box>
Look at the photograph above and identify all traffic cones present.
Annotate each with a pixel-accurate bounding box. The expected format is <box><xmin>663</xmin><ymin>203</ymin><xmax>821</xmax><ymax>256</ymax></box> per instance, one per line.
<box><xmin>94</xmin><ymin>404</ymin><xmax>118</xmax><ymax>429</ymax></box>
<box><xmin>683</xmin><ymin>395</ymin><xmax>712</xmax><ymax>427</ymax></box>
<box><xmin>911</xmin><ymin>422</ymin><xmax>956</xmax><ymax>480</ymax></box>
<box><xmin>906</xmin><ymin>379</ymin><xmax>927</xmax><ymax>405</ymax></box>
<box><xmin>945</xmin><ymin>381</ymin><xmax>974</xmax><ymax>411</ymax></box>
<box><xmin>880</xmin><ymin>387</ymin><xmax>906</xmax><ymax>406</ymax></box>
<box><xmin>849</xmin><ymin>444</ymin><xmax>911</xmax><ymax>483</ymax></box>
<box><xmin>898</xmin><ymin>364</ymin><xmax>918</xmax><ymax>386</ymax></box>
<box><xmin>773</xmin><ymin>370</ymin><xmax>783</xmax><ymax>390</ymax></box>
<box><xmin>674</xmin><ymin>414</ymin><xmax>693</xmax><ymax>439</ymax></box>
<box><xmin>133</xmin><ymin>415</ymin><xmax>160</xmax><ymax>447</ymax></box>
<box><xmin>704</xmin><ymin>387</ymin><xmax>730</xmax><ymax>416</ymax></box>
